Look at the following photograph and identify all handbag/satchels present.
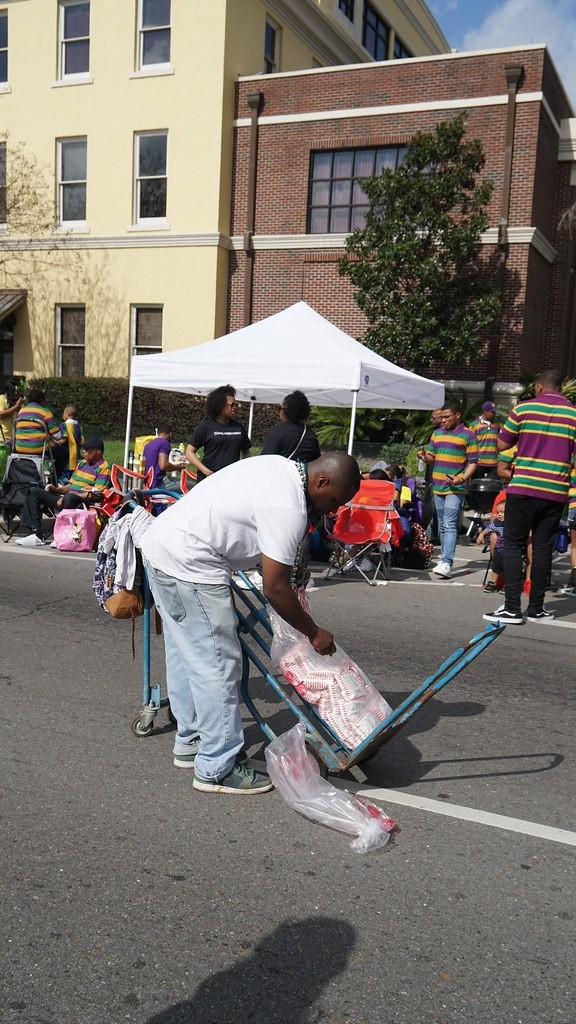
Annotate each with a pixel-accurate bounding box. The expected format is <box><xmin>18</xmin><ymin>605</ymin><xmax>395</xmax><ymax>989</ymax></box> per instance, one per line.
<box><xmin>53</xmin><ymin>503</ymin><xmax>96</xmax><ymax>552</ymax></box>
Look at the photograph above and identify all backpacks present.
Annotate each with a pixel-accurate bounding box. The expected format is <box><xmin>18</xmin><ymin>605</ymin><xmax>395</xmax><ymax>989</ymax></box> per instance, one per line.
<box><xmin>93</xmin><ymin>488</ymin><xmax>155</xmax><ymax>619</ymax></box>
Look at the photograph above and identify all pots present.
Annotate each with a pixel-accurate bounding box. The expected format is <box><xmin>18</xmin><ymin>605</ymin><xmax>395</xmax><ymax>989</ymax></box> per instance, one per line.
<box><xmin>465</xmin><ymin>472</ymin><xmax>503</xmax><ymax>513</ymax></box>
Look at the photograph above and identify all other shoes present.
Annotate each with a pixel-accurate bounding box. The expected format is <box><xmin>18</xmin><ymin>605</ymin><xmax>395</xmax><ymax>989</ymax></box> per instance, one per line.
<box><xmin>328</xmin><ymin>549</ymin><xmax>383</xmax><ymax>572</ymax></box>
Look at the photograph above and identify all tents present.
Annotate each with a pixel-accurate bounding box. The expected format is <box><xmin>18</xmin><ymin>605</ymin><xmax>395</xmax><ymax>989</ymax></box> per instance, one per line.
<box><xmin>122</xmin><ymin>301</ymin><xmax>445</xmax><ymax>499</ymax></box>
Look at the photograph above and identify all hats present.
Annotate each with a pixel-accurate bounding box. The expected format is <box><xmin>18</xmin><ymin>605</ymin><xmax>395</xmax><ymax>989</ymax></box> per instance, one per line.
<box><xmin>79</xmin><ymin>435</ymin><xmax>104</xmax><ymax>449</ymax></box>
<box><xmin>482</xmin><ymin>401</ymin><xmax>499</xmax><ymax>412</ymax></box>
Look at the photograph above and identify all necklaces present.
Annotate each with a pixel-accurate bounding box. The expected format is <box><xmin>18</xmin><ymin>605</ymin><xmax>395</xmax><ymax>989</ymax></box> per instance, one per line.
<box><xmin>290</xmin><ymin>459</ymin><xmax>311</xmax><ymax>591</ymax></box>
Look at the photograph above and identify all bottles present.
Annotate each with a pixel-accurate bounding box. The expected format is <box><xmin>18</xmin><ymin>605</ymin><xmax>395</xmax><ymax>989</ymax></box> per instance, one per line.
<box><xmin>179</xmin><ymin>443</ymin><xmax>185</xmax><ymax>452</ymax></box>
<box><xmin>418</xmin><ymin>442</ymin><xmax>424</xmax><ymax>472</ymax></box>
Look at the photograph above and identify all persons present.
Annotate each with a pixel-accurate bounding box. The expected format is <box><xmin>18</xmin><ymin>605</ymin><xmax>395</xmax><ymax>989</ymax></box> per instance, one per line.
<box><xmin>143</xmin><ymin>424</ymin><xmax>188</xmax><ymax>515</ymax></box>
<box><xmin>482</xmin><ymin>369</ymin><xmax>576</xmax><ymax>624</ymax></box>
<box><xmin>186</xmin><ymin>385</ymin><xmax>252</xmax><ymax>486</ymax></box>
<box><xmin>14</xmin><ymin>389</ymin><xmax>69</xmax><ymax>491</ymax></box>
<box><xmin>261</xmin><ymin>391</ymin><xmax>321</xmax><ymax>588</ymax></box>
<box><xmin>141</xmin><ymin>450</ymin><xmax>361</xmax><ymax>793</ymax></box>
<box><xmin>17</xmin><ymin>436</ymin><xmax>113</xmax><ymax>548</ymax></box>
<box><xmin>59</xmin><ymin>405</ymin><xmax>84</xmax><ymax>480</ymax></box>
<box><xmin>361</xmin><ymin>401</ymin><xmax>576</xmax><ymax>596</ymax></box>
<box><xmin>0</xmin><ymin>380</ymin><xmax>27</xmax><ymax>452</ymax></box>
<box><xmin>417</xmin><ymin>401</ymin><xmax>478</xmax><ymax>577</ymax></box>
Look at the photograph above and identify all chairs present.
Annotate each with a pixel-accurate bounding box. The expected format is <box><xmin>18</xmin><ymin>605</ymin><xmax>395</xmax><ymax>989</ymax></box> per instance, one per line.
<box><xmin>323</xmin><ymin>479</ymin><xmax>404</xmax><ymax>587</ymax></box>
<box><xmin>0</xmin><ymin>455</ymin><xmax>47</xmax><ymax>544</ymax></box>
<box><xmin>41</xmin><ymin>489</ymin><xmax>101</xmax><ymax>542</ymax></box>
<box><xmin>88</xmin><ymin>462</ymin><xmax>154</xmax><ymax>553</ymax></box>
<box><xmin>395</xmin><ymin>476</ymin><xmax>423</xmax><ymax>523</ymax></box>
<box><xmin>1</xmin><ymin>417</ymin><xmax>61</xmax><ymax>487</ymax></box>
<box><xmin>166</xmin><ymin>469</ymin><xmax>197</xmax><ymax>504</ymax></box>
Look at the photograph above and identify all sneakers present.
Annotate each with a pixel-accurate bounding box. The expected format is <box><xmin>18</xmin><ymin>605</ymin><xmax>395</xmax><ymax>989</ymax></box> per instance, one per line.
<box><xmin>483</xmin><ymin>602</ymin><xmax>523</xmax><ymax>624</ymax></box>
<box><xmin>526</xmin><ymin>605</ymin><xmax>554</xmax><ymax>622</ymax></box>
<box><xmin>232</xmin><ymin>569</ymin><xmax>263</xmax><ymax>591</ymax></box>
<box><xmin>15</xmin><ymin>532</ymin><xmax>45</xmax><ymax>546</ymax></box>
<box><xmin>484</xmin><ymin>581</ymin><xmax>497</xmax><ymax>592</ymax></box>
<box><xmin>50</xmin><ymin>540</ymin><xmax>57</xmax><ymax>548</ymax></box>
<box><xmin>499</xmin><ymin>586</ymin><xmax>505</xmax><ymax>594</ymax></box>
<box><xmin>173</xmin><ymin>745</ymin><xmax>248</xmax><ymax>769</ymax></box>
<box><xmin>192</xmin><ymin>758</ymin><xmax>273</xmax><ymax>795</ymax></box>
<box><xmin>432</xmin><ymin>558</ymin><xmax>451</xmax><ymax>578</ymax></box>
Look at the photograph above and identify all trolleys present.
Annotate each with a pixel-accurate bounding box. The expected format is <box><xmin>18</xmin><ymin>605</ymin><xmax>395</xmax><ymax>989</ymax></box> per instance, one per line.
<box><xmin>126</xmin><ymin>488</ymin><xmax>507</xmax><ymax>788</ymax></box>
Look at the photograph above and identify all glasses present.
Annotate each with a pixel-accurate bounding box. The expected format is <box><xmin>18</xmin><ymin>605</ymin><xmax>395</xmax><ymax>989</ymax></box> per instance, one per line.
<box><xmin>81</xmin><ymin>448</ymin><xmax>94</xmax><ymax>453</ymax></box>
<box><xmin>439</xmin><ymin>412</ymin><xmax>457</xmax><ymax>421</ymax></box>
<box><xmin>226</xmin><ymin>402</ymin><xmax>238</xmax><ymax>408</ymax></box>
<box><xmin>278</xmin><ymin>405</ymin><xmax>285</xmax><ymax>413</ymax></box>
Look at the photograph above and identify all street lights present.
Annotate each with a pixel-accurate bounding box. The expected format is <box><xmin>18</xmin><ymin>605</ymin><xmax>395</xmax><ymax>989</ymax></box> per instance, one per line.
<box><xmin>479</xmin><ymin>60</ymin><xmax>527</xmax><ymax>400</ymax></box>
<box><xmin>244</xmin><ymin>88</ymin><xmax>267</xmax><ymax>327</ymax></box>
